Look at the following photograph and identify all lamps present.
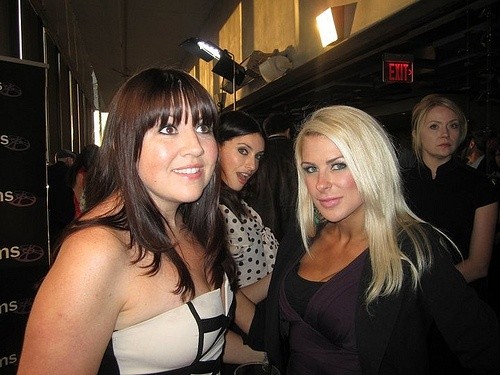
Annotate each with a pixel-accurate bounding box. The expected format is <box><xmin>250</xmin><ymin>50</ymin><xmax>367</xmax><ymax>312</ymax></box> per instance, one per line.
<box><xmin>180</xmin><ymin>37</ymin><xmax>246</xmax><ymax>111</ymax></box>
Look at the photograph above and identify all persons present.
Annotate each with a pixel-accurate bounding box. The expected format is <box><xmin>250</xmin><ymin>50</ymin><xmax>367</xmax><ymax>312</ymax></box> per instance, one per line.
<box><xmin>71</xmin><ymin>144</ymin><xmax>100</xmax><ymax>220</ymax></box>
<box><xmin>16</xmin><ymin>68</ymin><xmax>269</xmax><ymax>374</ymax></box>
<box><xmin>393</xmin><ymin>132</ymin><xmax>500</xmax><ymax>190</ymax></box>
<box><xmin>401</xmin><ymin>95</ymin><xmax>499</xmax><ymax>321</ymax></box>
<box><xmin>47</xmin><ymin>149</ymin><xmax>76</xmax><ymax>266</ymax></box>
<box><xmin>212</xmin><ymin>110</ymin><xmax>280</xmax><ymax>313</ymax></box>
<box><xmin>233</xmin><ymin>104</ymin><xmax>500</xmax><ymax>375</ymax></box>
<box><xmin>255</xmin><ymin>113</ymin><xmax>299</xmax><ymax>245</ymax></box>
